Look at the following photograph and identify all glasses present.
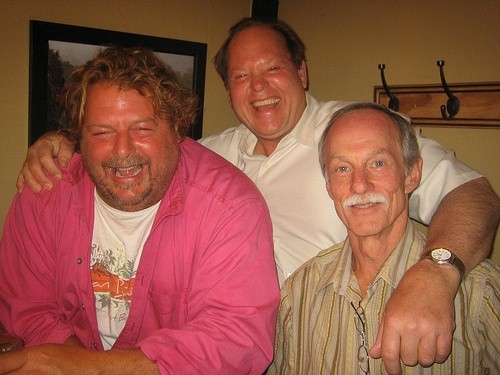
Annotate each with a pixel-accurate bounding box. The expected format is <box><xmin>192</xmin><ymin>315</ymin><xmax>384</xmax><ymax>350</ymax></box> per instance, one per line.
<box><xmin>350</xmin><ymin>301</ymin><xmax>370</xmax><ymax>375</ymax></box>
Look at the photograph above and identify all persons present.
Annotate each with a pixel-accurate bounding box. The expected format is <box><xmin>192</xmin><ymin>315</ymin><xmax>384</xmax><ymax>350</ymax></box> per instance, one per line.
<box><xmin>0</xmin><ymin>40</ymin><xmax>283</xmax><ymax>375</ymax></box>
<box><xmin>14</xmin><ymin>8</ymin><xmax>500</xmax><ymax>375</ymax></box>
<box><xmin>260</xmin><ymin>102</ymin><xmax>500</xmax><ymax>375</ymax></box>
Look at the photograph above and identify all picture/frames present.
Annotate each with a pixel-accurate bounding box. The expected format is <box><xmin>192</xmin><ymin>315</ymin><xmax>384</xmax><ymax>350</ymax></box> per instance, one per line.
<box><xmin>27</xmin><ymin>19</ymin><xmax>207</xmax><ymax>149</ymax></box>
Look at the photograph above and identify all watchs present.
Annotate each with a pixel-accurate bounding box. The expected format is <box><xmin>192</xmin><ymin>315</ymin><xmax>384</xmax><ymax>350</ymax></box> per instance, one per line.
<box><xmin>420</xmin><ymin>247</ymin><xmax>465</xmax><ymax>280</ymax></box>
